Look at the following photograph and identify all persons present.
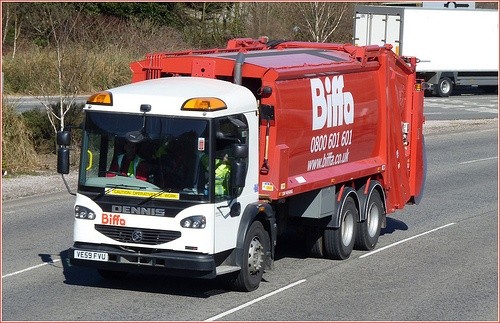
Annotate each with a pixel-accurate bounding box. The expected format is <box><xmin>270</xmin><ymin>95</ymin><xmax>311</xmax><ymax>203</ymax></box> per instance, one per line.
<box><xmin>109</xmin><ymin>141</ymin><xmax>148</xmax><ymax>181</ymax></box>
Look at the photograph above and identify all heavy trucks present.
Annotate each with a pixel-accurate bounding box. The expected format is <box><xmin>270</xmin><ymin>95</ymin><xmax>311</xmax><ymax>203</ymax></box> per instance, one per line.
<box><xmin>56</xmin><ymin>36</ymin><xmax>428</xmax><ymax>292</ymax></box>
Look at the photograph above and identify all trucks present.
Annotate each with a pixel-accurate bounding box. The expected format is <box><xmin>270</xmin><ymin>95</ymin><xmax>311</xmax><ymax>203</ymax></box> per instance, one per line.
<box><xmin>353</xmin><ymin>5</ymin><xmax>500</xmax><ymax>98</ymax></box>
